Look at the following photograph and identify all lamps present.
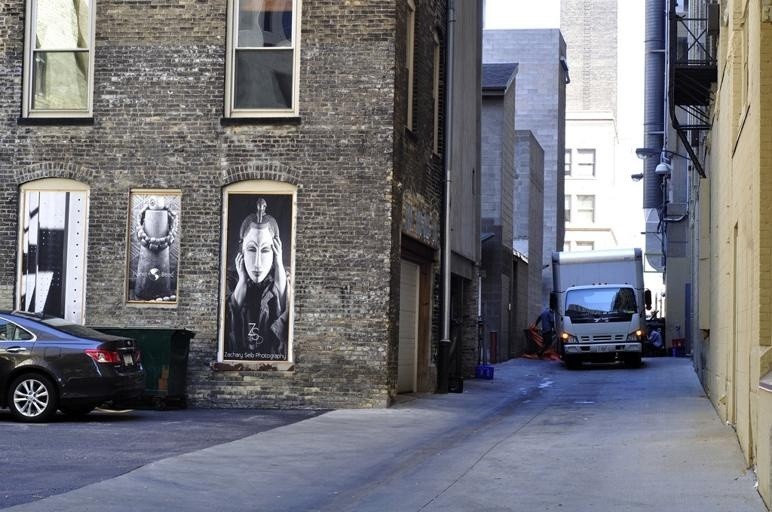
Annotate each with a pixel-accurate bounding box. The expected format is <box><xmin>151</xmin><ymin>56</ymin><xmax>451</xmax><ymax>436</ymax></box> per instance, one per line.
<box><xmin>632</xmin><ymin>173</ymin><xmax>661</xmax><ymax>181</ymax></box>
<box><xmin>636</xmin><ymin>147</ymin><xmax>690</xmax><ymax>161</ymax></box>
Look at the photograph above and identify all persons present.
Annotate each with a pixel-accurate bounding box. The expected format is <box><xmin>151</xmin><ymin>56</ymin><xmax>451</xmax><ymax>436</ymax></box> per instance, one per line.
<box><xmin>223</xmin><ymin>195</ymin><xmax>290</xmax><ymax>362</ymax></box>
<box><xmin>535</xmin><ymin>307</ymin><xmax>554</xmax><ymax>360</ymax></box>
<box><xmin>645</xmin><ymin>324</ymin><xmax>664</xmax><ymax>349</ymax></box>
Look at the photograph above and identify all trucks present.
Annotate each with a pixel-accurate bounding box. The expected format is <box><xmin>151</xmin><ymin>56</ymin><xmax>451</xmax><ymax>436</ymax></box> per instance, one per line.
<box><xmin>548</xmin><ymin>247</ymin><xmax>652</xmax><ymax>369</ymax></box>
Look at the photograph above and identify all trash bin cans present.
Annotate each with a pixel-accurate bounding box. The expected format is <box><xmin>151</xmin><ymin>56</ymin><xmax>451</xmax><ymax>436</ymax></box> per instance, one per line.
<box><xmin>85</xmin><ymin>327</ymin><xmax>195</xmax><ymax>411</ymax></box>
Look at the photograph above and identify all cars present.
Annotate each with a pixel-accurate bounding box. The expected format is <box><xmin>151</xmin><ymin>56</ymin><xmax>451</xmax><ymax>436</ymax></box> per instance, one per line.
<box><xmin>1</xmin><ymin>310</ymin><xmax>148</xmax><ymax>423</ymax></box>
